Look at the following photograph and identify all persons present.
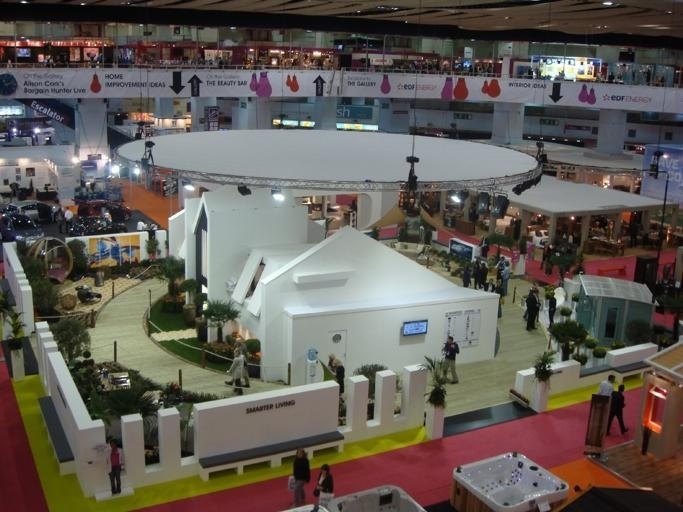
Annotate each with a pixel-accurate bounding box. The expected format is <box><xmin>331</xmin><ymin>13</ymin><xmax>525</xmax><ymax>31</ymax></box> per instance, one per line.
<box><xmin>56</xmin><ymin>207</ymin><xmax>64</xmax><ymax>234</ymax></box>
<box><xmin>313</xmin><ymin>463</ymin><xmax>333</xmax><ymax>493</ymax></box>
<box><xmin>478</xmin><ymin>235</ymin><xmax>485</xmax><ymax>256</ymax></box>
<box><xmin>628</xmin><ymin>219</ymin><xmax>639</xmax><ymax>249</ymax></box>
<box><xmin>441</xmin><ymin>336</ymin><xmax>459</xmax><ymax>383</ymax></box>
<box><xmin>483</xmin><ymin>238</ymin><xmax>490</xmax><ymax>257</ymax></box>
<box><xmin>606</xmin><ymin>383</ymin><xmax>628</xmax><ymax>435</ymax></box>
<box><xmin>135</xmin><ymin>218</ymin><xmax>147</xmax><ymax>232</ymax></box>
<box><xmin>64</xmin><ymin>207</ymin><xmax>73</xmax><ymax>233</ymax></box>
<box><xmin>597</xmin><ymin>374</ymin><xmax>616</xmax><ymax>396</ymax></box>
<box><xmin>606</xmin><ymin>220</ymin><xmax>616</xmax><ymax>241</ymax></box>
<box><xmin>292</xmin><ymin>446</ymin><xmax>310</xmax><ymax>507</ymax></box>
<box><xmin>327</xmin><ymin>353</ymin><xmax>336</xmax><ymax>372</ymax></box>
<box><xmin>227</xmin><ymin>348</ymin><xmax>242</xmax><ymax>395</ymax></box>
<box><xmin>332</xmin><ymin>358</ymin><xmax>344</xmax><ymax>395</ymax></box>
<box><xmin>463</xmin><ymin>255</ymin><xmax>540</xmax><ymax>332</ymax></box>
<box><xmin>545</xmin><ymin>291</ymin><xmax>556</xmax><ymax>328</ymax></box>
<box><xmin>518</xmin><ymin>227</ymin><xmax>581</xmax><ymax>280</ymax></box>
<box><xmin>0</xmin><ymin>47</ymin><xmax>683</xmax><ymax>88</ymax></box>
<box><xmin>105</xmin><ymin>439</ymin><xmax>122</xmax><ymax>494</ymax></box>
<box><xmin>225</xmin><ymin>343</ymin><xmax>250</xmax><ymax>387</ymax></box>
<box><xmin>235</xmin><ymin>336</ymin><xmax>246</xmax><ymax>354</ymax></box>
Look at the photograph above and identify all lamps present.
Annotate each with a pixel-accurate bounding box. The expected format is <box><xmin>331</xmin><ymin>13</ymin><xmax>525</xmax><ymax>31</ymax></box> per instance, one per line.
<box><xmin>398</xmin><ymin>4</ymin><xmax>421</xmax><ymax>219</ymax></box>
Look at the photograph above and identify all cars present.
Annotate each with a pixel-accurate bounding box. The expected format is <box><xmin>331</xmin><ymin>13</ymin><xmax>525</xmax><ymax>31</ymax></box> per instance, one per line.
<box><xmin>3</xmin><ymin>199</ymin><xmax>65</xmax><ymax>227</ymax></box>
<box><xmin>78</xmin><ymin>198</ymin><xmax>132</xmax><ymax>222</ymax></box>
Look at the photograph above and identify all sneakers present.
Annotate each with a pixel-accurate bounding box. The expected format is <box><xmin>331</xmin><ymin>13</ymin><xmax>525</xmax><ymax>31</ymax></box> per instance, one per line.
<box><xmin>451</xmin><ymin>380</ymin><xmax>458</xmax><ymax>384</ymax></box>
<box><xmin>622</xmin><ymin>428</ymin><xmax>628</xmax><ymax>434</ymax></box>
<box><xmin>225</xmin><ymin>381</ymin><xmax>232</xmax><ymax>385</ymax></box>
<box><xmin>243</xmin><ymin>385</ymin><xmax>249</xmax><ymax>387</ymax></box>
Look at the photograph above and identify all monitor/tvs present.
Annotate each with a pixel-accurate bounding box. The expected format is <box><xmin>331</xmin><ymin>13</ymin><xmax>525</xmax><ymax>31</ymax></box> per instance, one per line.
<box><xmin>402</xmin><ymin>319</ymin><xmax>428</xmax><ymax>336</ymax></box>
